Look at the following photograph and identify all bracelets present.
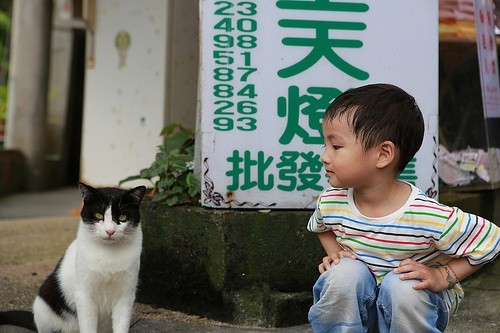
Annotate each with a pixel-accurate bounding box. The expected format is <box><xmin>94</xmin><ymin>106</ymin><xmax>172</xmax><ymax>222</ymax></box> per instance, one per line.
<box><xmin>447</xmin><ymin>262</ymin><xmax>460</xmax><ymax>284</ymax></box>
<box><xmin>442</xmin><ymin>265</ymin><xmax>455</xmax><ymax>288</ymax></box>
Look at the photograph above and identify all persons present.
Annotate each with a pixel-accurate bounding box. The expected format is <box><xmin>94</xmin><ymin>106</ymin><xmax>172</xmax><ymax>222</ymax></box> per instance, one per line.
<box><xmin>306</xmin><ymin>84</ymin><xmax>499</xmax><ymax>333</ymax></box>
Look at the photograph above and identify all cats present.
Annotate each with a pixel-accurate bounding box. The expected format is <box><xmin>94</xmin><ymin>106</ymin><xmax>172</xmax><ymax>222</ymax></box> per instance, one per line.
<box><xmin>0</xmin><ymin>182</ymin><xmax>147</xmax><ymax>333</ymax></box>
